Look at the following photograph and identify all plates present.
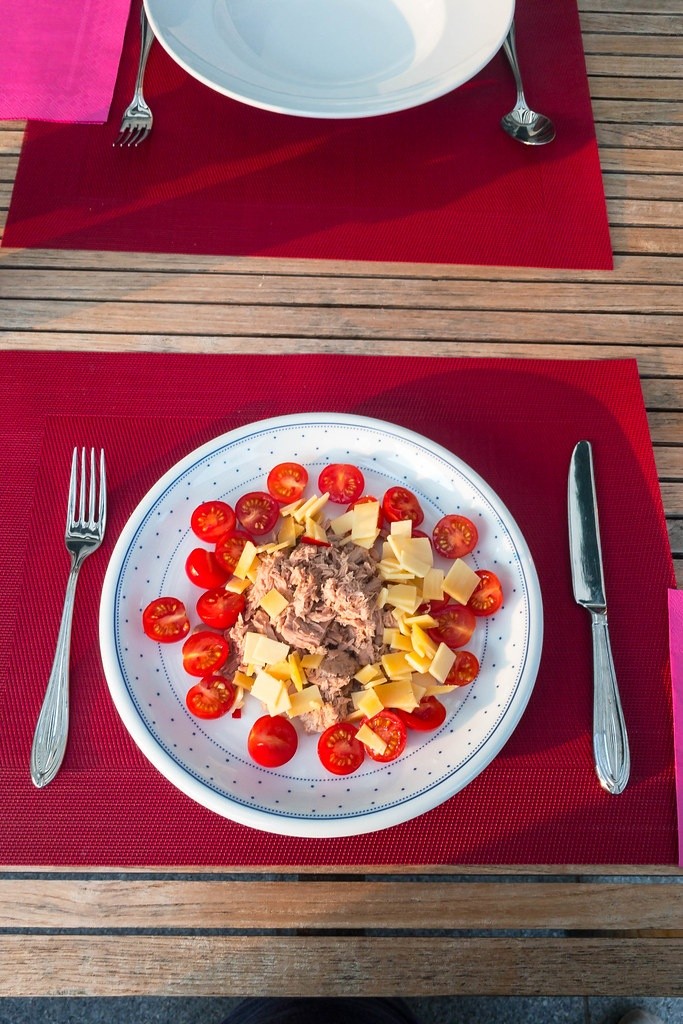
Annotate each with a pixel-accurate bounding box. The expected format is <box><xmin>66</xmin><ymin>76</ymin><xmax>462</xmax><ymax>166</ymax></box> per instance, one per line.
<box><xmin>131</xmin><ymin>1</ymin><xmax>520</xmax><ymax>118</ymax></box>
<box><xmin>101</xmin><ymin>412</ymin><xmax>542</xmax><ymax>841</ymax></box>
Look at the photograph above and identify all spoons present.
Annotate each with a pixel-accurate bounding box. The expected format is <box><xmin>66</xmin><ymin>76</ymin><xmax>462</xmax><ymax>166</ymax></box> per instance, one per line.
<box><xmin>500</xmin><ymin>23</ymin><xmax>555</xmax><ymax>143</ymax></box>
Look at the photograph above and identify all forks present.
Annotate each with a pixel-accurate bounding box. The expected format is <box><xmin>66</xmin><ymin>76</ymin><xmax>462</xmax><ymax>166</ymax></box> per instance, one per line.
<box><xmin>28</xmin><ymin>447</ymin><xmax>109</xmax><ymax>787</ymax></box>
<box><xmin>116</xmin><ymin>8</ymin><xmax>184</xmax><ymax>146</ymax></box>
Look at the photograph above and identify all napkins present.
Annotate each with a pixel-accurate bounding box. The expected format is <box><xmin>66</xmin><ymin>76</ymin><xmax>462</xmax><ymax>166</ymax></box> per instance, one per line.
<box><xmin>0</xmin><ymin>0</ymin><xmax>131</xmax><ymax>125</ymax></box>
<box><xmin>668</xmin><ymin>589</ymin><xmax>683</xmax><ymax>868</ymax></box>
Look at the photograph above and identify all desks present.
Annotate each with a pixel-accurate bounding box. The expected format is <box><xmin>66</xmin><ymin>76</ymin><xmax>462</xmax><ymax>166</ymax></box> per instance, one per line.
<box><xmin>0</xmin><ymin>0</ymin><xmax>683</xmax><ymax>997</ymax></box>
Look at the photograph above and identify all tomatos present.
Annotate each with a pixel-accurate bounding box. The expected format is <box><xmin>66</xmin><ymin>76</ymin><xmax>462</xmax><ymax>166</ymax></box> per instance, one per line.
<box><xmin>143</xmin><ymin>462</ymin><xmax>503</xmax><ymax>775</ymax></box>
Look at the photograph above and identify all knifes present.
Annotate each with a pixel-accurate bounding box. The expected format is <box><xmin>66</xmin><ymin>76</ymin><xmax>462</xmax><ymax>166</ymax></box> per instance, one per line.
<box><xmin>567</xmin><ymin>440</ymin><xmax>630</xmax><ymax>796</ymax></box>
